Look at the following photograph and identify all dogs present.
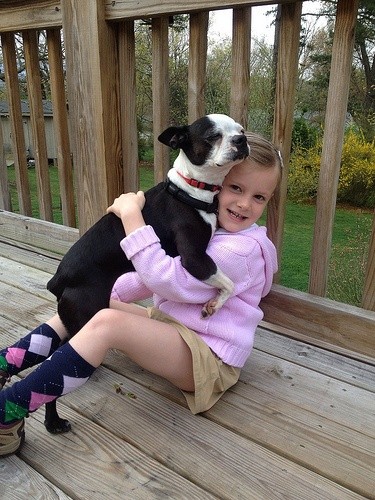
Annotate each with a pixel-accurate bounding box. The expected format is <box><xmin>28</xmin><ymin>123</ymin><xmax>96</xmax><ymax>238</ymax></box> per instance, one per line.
<box><xmin>44</xmin><ymin>113</ymin><xmax>251</xmax><ymax>435</ymax></box>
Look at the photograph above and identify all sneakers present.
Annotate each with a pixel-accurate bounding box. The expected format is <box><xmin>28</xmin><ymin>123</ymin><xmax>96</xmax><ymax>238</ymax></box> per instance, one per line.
<box><xmin>0</xmin><ymin>417</ymin><xmax>26</xmax><ymax>456</ymax></box>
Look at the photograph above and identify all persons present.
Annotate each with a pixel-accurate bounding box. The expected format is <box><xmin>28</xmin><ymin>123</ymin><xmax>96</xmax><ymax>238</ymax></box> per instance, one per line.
<box><xmin>0</xmin><ymin>131</ymin><xmax>284</xmax><ymax>455</ymax></box>
